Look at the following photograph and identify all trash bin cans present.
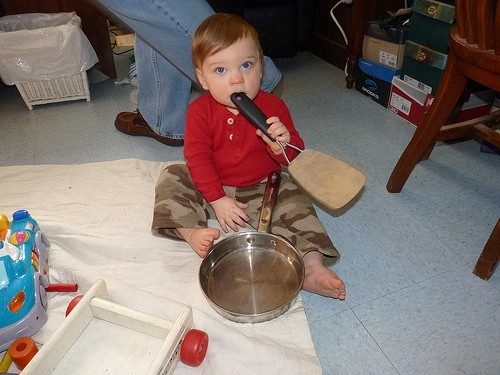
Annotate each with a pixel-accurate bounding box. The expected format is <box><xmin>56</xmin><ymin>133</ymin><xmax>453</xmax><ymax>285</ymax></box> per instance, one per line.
<box><xmin>0</xmin><ymin>10</ymin><xmax>100</xmax><ymax>112</ymax></box>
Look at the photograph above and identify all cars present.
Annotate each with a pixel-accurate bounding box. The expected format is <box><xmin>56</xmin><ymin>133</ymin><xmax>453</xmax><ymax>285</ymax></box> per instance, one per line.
<box><xmin>0</xmin><ymin>209</ymin><xmax>50</xmax><ymax>353</ymax></box>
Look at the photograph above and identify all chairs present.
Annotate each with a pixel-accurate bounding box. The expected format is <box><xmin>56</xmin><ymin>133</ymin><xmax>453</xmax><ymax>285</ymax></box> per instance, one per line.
<box><xmin>387</xmin><ymin>0</ymin><xmax>500</xmax><ymax>280</ymax></box>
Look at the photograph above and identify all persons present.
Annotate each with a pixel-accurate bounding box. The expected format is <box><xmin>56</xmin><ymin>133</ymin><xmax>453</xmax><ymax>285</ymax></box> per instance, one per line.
<box><xmin>98</xmin><ymin>0</ymin><xmax>281</xmax><ymax>148</ymax></box>
<box><xmin>150</xmin><ymin>13</ymin><xmax>346</xmax><ymax>300</ymax></box>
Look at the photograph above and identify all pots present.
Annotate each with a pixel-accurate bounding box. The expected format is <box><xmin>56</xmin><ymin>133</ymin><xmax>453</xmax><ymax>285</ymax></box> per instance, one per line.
<box><xmin>199</xmin><ymin>170</ymin><xmax>305</xmax><ymax>323</ymax></box>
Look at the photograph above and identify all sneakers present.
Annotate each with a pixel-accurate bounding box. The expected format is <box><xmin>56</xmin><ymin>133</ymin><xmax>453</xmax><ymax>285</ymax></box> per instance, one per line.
<box><xmin>271</xmin><ymin>77</ymin><xmax>284</xmax><ymax>98</ymax></box>
<box><xmin>114</xmin><ymin>112</ymin><xmax>185</xmax><ymax>147</ymax></box>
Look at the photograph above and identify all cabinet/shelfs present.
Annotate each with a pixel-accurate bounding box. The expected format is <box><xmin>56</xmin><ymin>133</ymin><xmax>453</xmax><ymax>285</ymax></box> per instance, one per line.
<box><xmin>306</xmin><ymin>0</ymin><xmax>388</xmax><ymax>88</ymax></box>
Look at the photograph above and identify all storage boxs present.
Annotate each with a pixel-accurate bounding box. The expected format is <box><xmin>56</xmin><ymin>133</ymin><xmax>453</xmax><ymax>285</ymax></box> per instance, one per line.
<box><xmin>362</xmin><ymin>20</ymin><xmax>410</xmax><ymax>69</ymax></box>
<box><xmin>400</xmin><ymin>40</ymin><xmax>448</xmax><ymax>95</ymax></box>
<box><xmin>0</xmin><ymin>12</ymin><xmax>91</xmax><ymax>111</ymax></box>
<box><xmin>387</xmin><ymin>75</ymin><xmax>436</xmax><ymax>125</ymax></box>
<box><xmin>355</xmin><ymin>57</ymin><xmax>401</xmax><ymax>108</ymax></box>
<box><xmin>406</xmin><ymin>0</ymin><xmax>455</xmax><ymax>54</ymax></box>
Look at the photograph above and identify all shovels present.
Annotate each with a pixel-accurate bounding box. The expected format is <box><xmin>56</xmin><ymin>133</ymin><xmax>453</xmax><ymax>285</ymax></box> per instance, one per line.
<box><xmin>230</xmin><ymin>92</ymin><xmax>366</xmax><ymax>211</ymax></box>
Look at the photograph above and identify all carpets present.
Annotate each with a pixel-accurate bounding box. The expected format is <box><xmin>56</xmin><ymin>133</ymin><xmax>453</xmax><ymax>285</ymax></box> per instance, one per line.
<box><xmin>1</xmin><ymin>158</ymin><xmax>322</xmax><ymax>375</ymax></box>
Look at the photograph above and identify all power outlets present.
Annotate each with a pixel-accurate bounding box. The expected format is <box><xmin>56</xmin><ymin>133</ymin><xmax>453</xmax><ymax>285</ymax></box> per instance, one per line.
<box><xmin>116</xmin><ymin>34</ymin><xmax>136</xmax><ymax>46</ymax></box>
